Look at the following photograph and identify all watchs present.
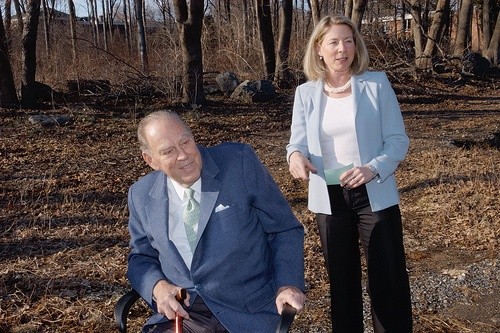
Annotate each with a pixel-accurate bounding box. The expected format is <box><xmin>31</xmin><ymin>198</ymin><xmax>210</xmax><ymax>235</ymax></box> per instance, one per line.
<box><xmin>366</xmin><ymin>165</ymin><xmax>379</xmax><ymax>176</ymax></box>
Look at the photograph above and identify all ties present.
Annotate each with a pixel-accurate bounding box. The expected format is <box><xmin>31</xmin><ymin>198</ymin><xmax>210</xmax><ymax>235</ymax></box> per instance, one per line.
<box><xmin>183</xmin><ymin>187</ymin><xmax>203</xmax><ymax>256</ymax></box>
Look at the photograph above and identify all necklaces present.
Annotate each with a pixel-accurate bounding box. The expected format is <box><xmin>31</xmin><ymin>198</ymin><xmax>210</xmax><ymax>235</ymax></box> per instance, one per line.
<box><xmin>323</xmin><ymin>76</ymin><xmax>351</xmax><ymax>94</ymax></box>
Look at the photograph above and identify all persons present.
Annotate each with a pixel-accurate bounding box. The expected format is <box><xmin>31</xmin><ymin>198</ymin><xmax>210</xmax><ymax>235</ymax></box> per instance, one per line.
<box><xmin>127</xmin><ymin>110</ymin><xmax>306</xmax><ymax>333</ymax></box>
<box><xmin>286</xmin><ymin>14</ymin><xmax>413</xmax><ymax>333</ymax></box>
